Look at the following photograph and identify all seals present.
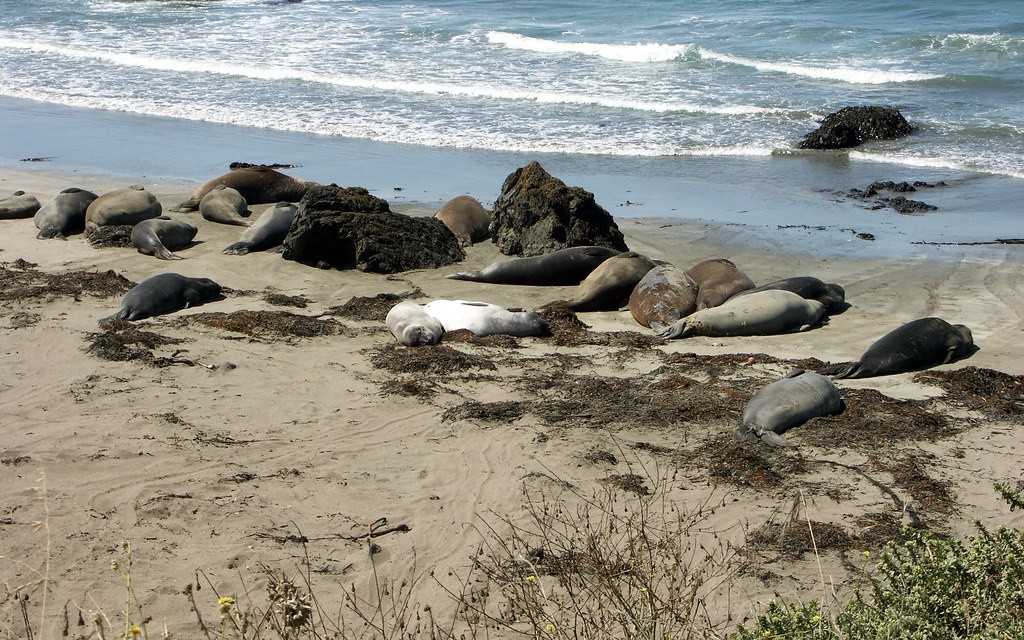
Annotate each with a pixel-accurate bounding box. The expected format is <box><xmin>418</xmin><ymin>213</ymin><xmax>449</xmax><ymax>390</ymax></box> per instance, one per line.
<box><xmin>130</xmin><ymin>216</ymin><xmax>199</xmax><ymax>261</ymax></box>
<box><xmin>422</xmin><ymin>299</ymin><xmax>551</xmax><ymax>337</ymax></box>
<box><xmin>815</xmin><ymin>317</ymin><xmax>973</xmax><ymax>379</ymax></box>
<box><xmin>220</xmin><ymin>200</ymin><xmax>299</xmax><ymax>256</ymax></box>
<box><xmin>435</xmin><ymin>195</ymin><xmax>494</xmax><ymax>249</ymax></box>
<box><xmin>168</xmin><ymin>165</ymin><xmax>324</xmax><ymax>213</ymax></box>
<box><xmin>386</xmin><ymin>300</ymin><xmax>443</xmax><ymax>347</ymax></box>
<box><xmin>33</xmin><ymin>188</ymin><xmax>100</xmax><ymax>242</ymax></box>
<box><xmin>444</xmin><ymin>246</ymin><xmax>621</xmax><ymax>286</ymax></box>
<box><xmin>83</xmin><ymin>183</ymin><xmax>163</xmax><ymax>240</ymax></box>
<box><xmin>199</xmin><ymin>184</ymin><xmax>254</xmax><ymax>228</ymax></box>
<box><xmin>117</xmin><ymin>273</ymin><xmax>222</xmax><ymax>321</ymax></box>
<box><xmin>733</xmin><ymin>368</ymin><xmax>840</xmax><ymax>449</ymax></box>
<box><xmin>0</xmin><ymin>191</ymin><xmax>41</xmax><ymax>220</ymax></box>
<box><xmin>565</xmin><ymin>252</ymin><xmax>845</xmax><ymax>341</ymax></box>
